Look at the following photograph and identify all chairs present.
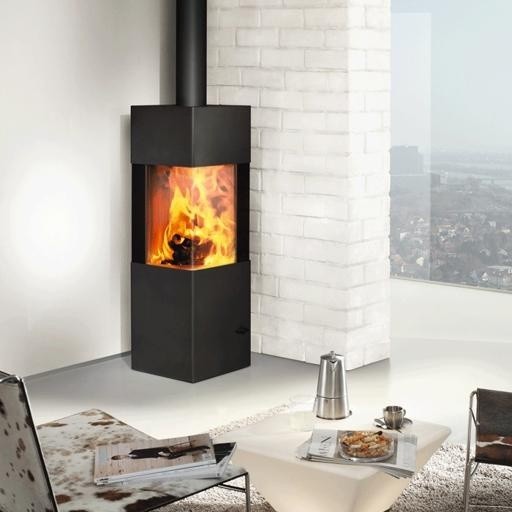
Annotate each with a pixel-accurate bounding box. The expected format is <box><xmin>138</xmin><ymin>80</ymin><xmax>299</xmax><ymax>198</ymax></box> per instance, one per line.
<box><xmin>0</xmin><ymin>371</ymin><xmax>251</xmax><ymax>512</ymax></box>
<box><xmin>463</xmin><ymin>388</ymin><xmax>512</xmax><ymax>512</ymax></box>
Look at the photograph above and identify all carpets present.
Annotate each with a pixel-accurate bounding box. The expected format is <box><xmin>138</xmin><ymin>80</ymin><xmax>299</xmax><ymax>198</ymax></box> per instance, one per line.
<box><xmin>148</xmin><ymin>394</ymin><xmax>512</xmax><ymax>512</ymax></box>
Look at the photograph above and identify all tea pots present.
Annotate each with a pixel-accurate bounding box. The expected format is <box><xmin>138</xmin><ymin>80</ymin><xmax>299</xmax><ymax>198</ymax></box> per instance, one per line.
<box><xmin>314</xmin><ymin>351</ymin><xmax>352</xmax><ymax>421</ymax></box>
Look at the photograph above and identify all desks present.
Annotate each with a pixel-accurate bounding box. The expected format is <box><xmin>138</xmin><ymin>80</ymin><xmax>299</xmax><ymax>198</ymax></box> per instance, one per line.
<box><xmin>213</xmin><ymin>395</ymin><xmax>452</xmax><ymax>512</ymax></box>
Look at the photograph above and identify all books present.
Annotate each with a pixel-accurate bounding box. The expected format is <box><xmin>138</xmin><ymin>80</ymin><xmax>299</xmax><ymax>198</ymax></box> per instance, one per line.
<box><xmin>93</xmin><ymin>432</ymin><xmax>237</xmax><ymax>485</ymax></box>
<box><xmin>296</xmin><ymin>430</ymin><xmax>417</xmax><ymax>479</ymax></box>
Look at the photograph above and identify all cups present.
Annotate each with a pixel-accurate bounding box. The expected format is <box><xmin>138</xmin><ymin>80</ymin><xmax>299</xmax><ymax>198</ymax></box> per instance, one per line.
<box><xmin>382</xmin><ymin>405</ymin><xmax>406</xmax><ymax>430</ymax></box>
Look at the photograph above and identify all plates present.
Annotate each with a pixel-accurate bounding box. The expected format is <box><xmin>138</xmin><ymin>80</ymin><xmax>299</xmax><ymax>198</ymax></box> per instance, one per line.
<box><xmin>337</xmin><ymin>431</ymin><xmax>395</xmax><ymax>463</ymax></box>
<box><xmin>375</xmin><ymin>419</ymin><xmax>413</xmax><ymax>433</ymax></box>
